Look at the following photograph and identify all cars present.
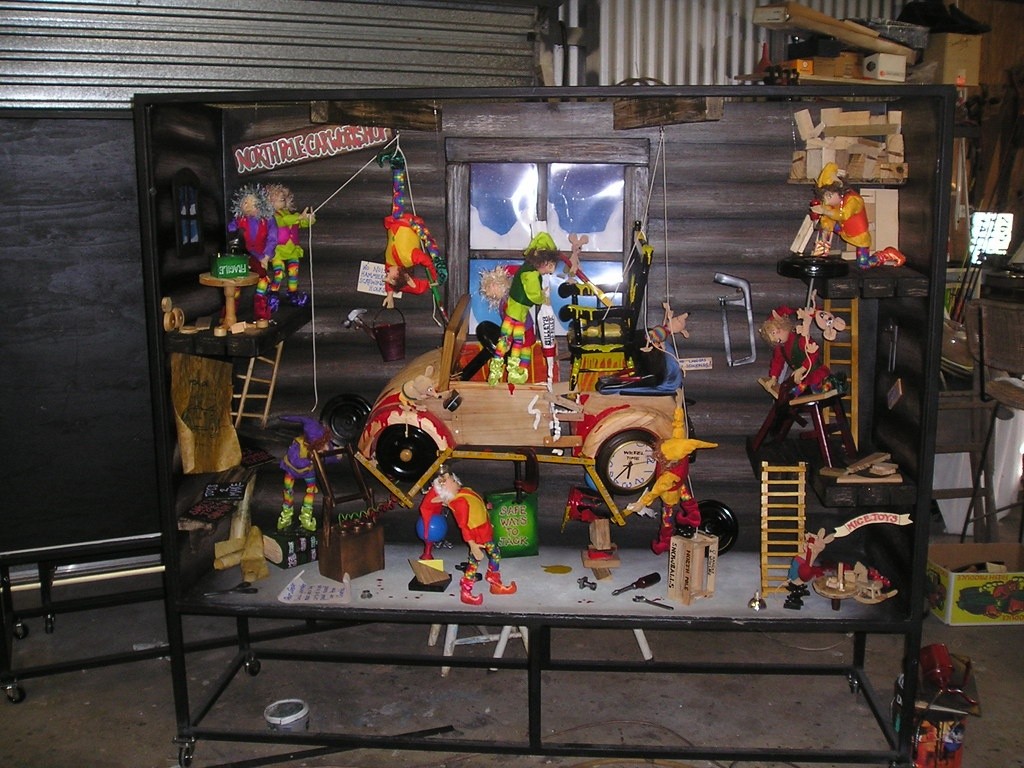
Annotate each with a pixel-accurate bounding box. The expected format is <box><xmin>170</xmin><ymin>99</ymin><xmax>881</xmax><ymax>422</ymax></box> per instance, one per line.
<box><xmin>355</xmin><ymin>288</ymin><xmax>695</xmax><ymax>495</ymax></box>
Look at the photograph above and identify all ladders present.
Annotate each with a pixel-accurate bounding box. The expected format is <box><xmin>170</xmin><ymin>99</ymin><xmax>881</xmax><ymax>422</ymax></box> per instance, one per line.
<box><xmin>823</xmin><ymin>298</ymin><xmax>858</xmax><ymax>451</ymax></box>
<box><xmin>761</xmin><ymin>461</ymin><xmax>806</xmax><ymax>597</ymax></box>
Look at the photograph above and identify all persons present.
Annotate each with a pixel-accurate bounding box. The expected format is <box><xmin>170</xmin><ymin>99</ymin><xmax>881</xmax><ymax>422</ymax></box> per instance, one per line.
<box><xmin>218</xmin><ymin>183</ymin><xmax>278</xmax><ymax>327</ymax></box>
<box><xmin>264</xmin><ymin>182</ymin><xmax>316</xmax><ymax>326</ymax></box>
<box><xmin>277</xmin><ymin>414</ymin><xmax>341</xmax><ymax>534</ymax></box>
<box><xmin>626</xmin><ymin>388</ymin><xmax>719</xmax><ymax>555</ymax></box>
<box><xmin>418</xmin><ymin>473</ymin><xmax>517</xmax><ymax>605</ymax></box>
<box><xmin>376</xmin><ymin>150</ymin><xmax>448</xmax><ymax>309</ymax></box>
<box><xmin>758</xmin><ymin>304</ymin><xmax>843</xmax><ymax>398</ymax></box>
<box><xmin>488</xmin><ymin>231</ymin><xmax>561</xmax><ymax>386</ymax></box>
<box><xmin>813</xmin><ymin>161</ymin><xmax>906</xmax><ymax>268</ymax></box>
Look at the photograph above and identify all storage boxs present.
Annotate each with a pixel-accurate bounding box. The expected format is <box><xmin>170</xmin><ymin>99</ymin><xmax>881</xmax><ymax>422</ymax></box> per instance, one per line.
<box><xmin>782</xmin><ymin>59</ymin><xmax>813</xmax><ymax>75</ymax></box>
<box><xmin>787</xmin><ymin>36</ymin><xmax>840</xmax><ymax>58</ymax></box>
<box><xmin>925</xmin><ymin>542</ymin><xmax>1024</xmax><ymax>626</ymax></box>
<box><xmin>811</xmin><ymin>57</ymin><xmax>843</xmax><ymax>77</ymax></box>
<box><xmin>835</xmin><ymin>53</ymin><xmax>864</xmax><ymax>77</ymax></box>
<box><xmin>862</xmin><ymin>53</ymin><xmax>907</xmax><ymax>82</ymax></box>
<box><xmin>923</xmin><ymin>33</ymin><xmax>983</xmax><ymax>86</ymax></box>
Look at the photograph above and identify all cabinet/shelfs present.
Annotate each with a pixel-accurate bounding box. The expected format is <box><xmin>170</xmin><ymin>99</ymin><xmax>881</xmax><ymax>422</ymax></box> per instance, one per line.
<box><xmin>125</xmin><ymin>71</ymin><xmax>1010</xmax><ymax>768</ymax></box>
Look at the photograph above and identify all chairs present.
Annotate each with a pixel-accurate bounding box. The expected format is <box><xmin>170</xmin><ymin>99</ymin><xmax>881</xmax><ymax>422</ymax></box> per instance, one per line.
<box><xmin>558</xmin><ymin>219</ymin><xmax>655</xmax><ymax>391</ymax></box>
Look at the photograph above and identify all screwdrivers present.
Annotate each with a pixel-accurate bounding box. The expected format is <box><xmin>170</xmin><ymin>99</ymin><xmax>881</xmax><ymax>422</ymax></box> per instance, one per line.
<box><xmin>612</xmin><ymin>572</ymin><xmax>661</xmax><ymax>597</ymax></box>
